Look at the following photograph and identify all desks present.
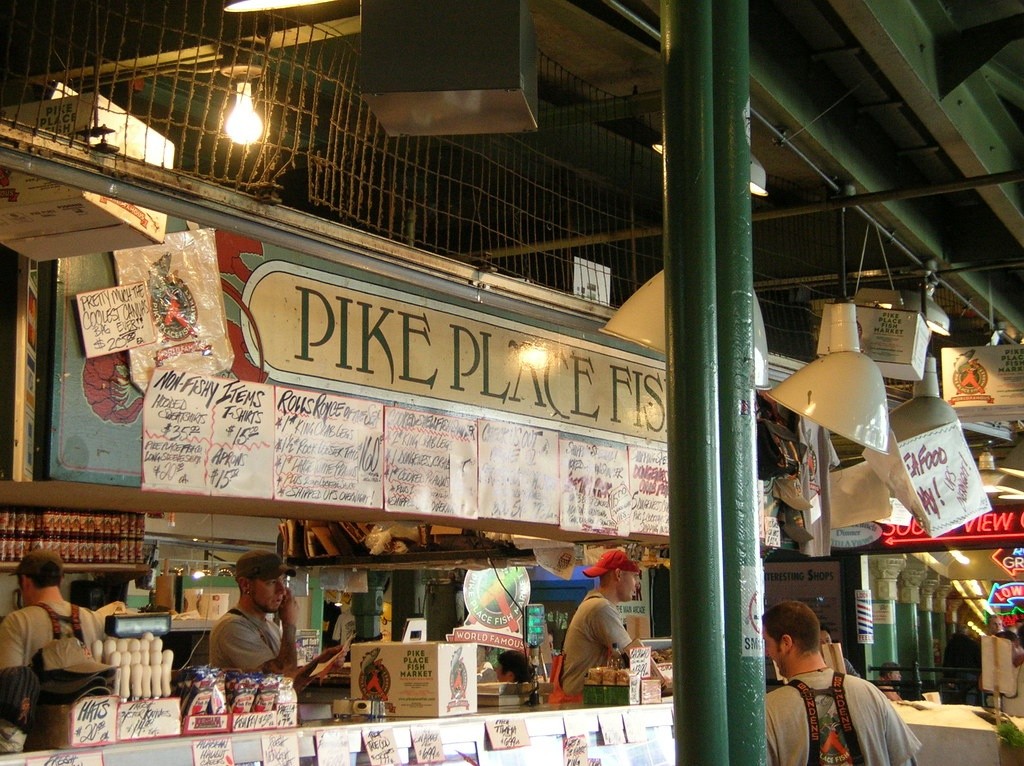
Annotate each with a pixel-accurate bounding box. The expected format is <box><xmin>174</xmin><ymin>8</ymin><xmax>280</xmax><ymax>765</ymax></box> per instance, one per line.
<box><xmin>890</xmin><ymin>701</ymin><xmax>1001</xmax><ymax>766</ymax></box>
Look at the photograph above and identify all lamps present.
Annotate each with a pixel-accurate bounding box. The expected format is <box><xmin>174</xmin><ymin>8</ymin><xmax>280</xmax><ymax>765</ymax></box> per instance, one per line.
<box><xmin>979</xmin><ymin>445</ymin><xmax>995</xmax><ymax>470</ymax></box>
<box><xmin>900</xmin><ymin>261</ymin><xmax>949</xmax><ymax>338</ymax></box>
<box><xmin>598</xmin><ymin>270</ymin><xmax>774</xmax><ymax>391</ymax></box>
<box><xmin>649</xmin><ymin>137</ymin><xmax>768</xmax><ymax>197</ymax></box>
<box><xmin>890</xmin><ymin>281</ymin><xmax>961</xmax><ymax>441</ymax></box>
<box><xmin>767</xmin><ymin>192</ymin><xmax>889</xmax><ymax>455</ymax></box>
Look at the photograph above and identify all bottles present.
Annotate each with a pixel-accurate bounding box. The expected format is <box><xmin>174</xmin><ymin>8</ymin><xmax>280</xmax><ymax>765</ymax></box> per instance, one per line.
<box><xmin>607</xmin><ymin>642</ymin><xmax>624</xmax><ymax>669</ymax></box>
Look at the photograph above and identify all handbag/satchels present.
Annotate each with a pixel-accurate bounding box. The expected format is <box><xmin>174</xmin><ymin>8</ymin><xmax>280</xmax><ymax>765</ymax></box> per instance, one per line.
<box><xmin>757</xmin><ymin>419</ymin><xmax>801</xmax><ymax>481</ymax></box>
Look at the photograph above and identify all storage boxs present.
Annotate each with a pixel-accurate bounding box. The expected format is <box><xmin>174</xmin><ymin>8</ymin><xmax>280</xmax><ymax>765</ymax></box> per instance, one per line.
<box><xmin>584</xmin><ymin>686</ymin><xmax>629</xmax><ymax>705</ymax></box>
<box><xmin>853</xmin><ymin>304</ymin><xmax>929</xmax><ymax>380</ymax></box>
<box><xmin>941</xmin><ymin>343</ymin><xmax>1024</xmax><ymax>425</ymax></box>
<box><xmin>623</xmin><ymin>637</ymin><xmax>673</xmax><ymax>694</ymax></box>
<box><xmin>42</xmin><ymin>693</ymin><xmax>297</xmax><ymax>748</ymax></box>
<box><xmin>1</xmin><ymin>83</ymin><xmax>175</xmax><ymax>261</ymax></box>
<box><xmin>351</xmin><ymin>642</ymin><xmax>478</xmax><ymax>717</ymax></box>
<box><xmin>552</xmin><ymin>257</ymin><xmax>610</xmax><ymax>305</ymax></box>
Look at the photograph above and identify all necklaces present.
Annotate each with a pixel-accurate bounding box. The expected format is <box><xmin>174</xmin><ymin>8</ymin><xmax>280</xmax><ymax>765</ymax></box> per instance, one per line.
<box><xmin>792</xmin><ymin>667</ymin><xmax>829</xmax><ymax>677</ymax></box>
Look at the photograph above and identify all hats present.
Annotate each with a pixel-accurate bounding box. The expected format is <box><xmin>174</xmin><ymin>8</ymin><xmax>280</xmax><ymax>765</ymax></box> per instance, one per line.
<box><xmin>30</xmin><ymin>636</ymin><xmax>117</xmax><ymax>708</ymax></box>
<box><xmin>236</xmin><ymin>551</ymin><xmax>297</xmax><ymax>582</ymax></box>
<box><xmin>582</xmin><ymin>551</ymin><xmax>640</xmax><ymax>578</ymax></box>
<box><xmin>8</xmin><ymin>550</ymin><xmax>64</xmax><ymax>577</ymax></box>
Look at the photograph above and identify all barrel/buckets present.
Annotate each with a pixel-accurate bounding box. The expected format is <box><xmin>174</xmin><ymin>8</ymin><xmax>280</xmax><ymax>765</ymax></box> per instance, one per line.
<box><xmin>181</xmin><ymin>588</ymin><xmax>203</xmax><ymax>614</ymax></box>
<box><xmin>198</xmin><ymin>593</ymin><xmax>229</xmax><ymax>620</ymax></box>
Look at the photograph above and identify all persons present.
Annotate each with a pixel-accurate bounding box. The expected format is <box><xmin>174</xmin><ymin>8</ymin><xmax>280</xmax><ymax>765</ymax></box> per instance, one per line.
<box><xmin>209</xmin><ymin>549</ymin><xmax>345</xmax><ymax>726</ymax></box>
<box><xmin>494</xmin><ymin>650</ymin><xmax>535</xmax><ymax>683</ymax></box>
<box><xmin>0</xmin><ymin>550</ymin><xmax>106</xmax><ymax>754</ymax></box>
<box><xmin>762</xmin><ymin>600</ymin><xmax>922</xmax><ymax>766</ymax></box>
<box><xmin>943</xmin><ymin>615</ymin><xmax>1024</xmax><ymax>718</ymax></box>
<box><xmin>548</xmin><ymin>548</ymin><xmax>640</xmax><ymax>704</ymax></box>
<box><xmin>875</xmin><ymin>662</ymin><xmax>903</xmax><ymax>702</ymax></box>
<box><xmin>819</xmin><ymin>622</ymin><xmax>861</xmax><ymax>678</ymax></box>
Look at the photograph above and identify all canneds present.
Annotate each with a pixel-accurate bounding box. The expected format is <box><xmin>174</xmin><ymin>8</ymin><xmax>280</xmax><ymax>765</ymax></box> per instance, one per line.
<box><xmin>0</xmin><ymin>506</ymin><xmax>146</xmax><ymax>562</ymax></box>
